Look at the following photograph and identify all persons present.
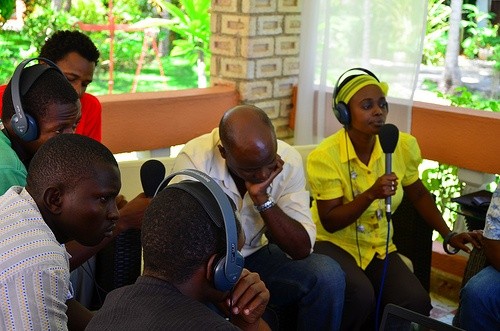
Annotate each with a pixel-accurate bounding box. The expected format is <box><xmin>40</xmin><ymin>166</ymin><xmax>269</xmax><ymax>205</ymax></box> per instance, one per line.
<box><xmin>0</xmin><ymin>58</ymin><xmax>156</xmax><ymax>311</ymax></box>
<box><xmin>306</xmin><ymin>68</ymin><xmax>486</xmax><ymax>331</ymax></box>
<box><xmin>0</xmin><ymin>30</ymin><xmax>103</xmax><ymax>144</ymax></box>
<box><xmin>0</xmin><ymin>132</ymin><xmax>122</xmax><ymax>331</ymax></box>
<box><xmin>459</xmin><ymin>182</ymin><xmax>500</xmax><ymax>331</ymax></box>
<box><xmin>168</xmin><ymin>105</ymin><xmax>346</xmax><ymax>331</ymax></box>
<box><xmin>84</xmin><ymin>168</ymin><xmax>272</xmax><ymax>331</ymax></box>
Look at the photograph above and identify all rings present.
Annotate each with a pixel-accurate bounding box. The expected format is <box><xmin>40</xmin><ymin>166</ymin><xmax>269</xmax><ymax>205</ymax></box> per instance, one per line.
<box><xmin>392</xmin><ymin>181</ymin><xmax>394</xmax><ymax>185</ymax></box>
<box><xmin>391</xmin><ymin>186</ymin><xmax>394</xmax><ymax>190</ymax></box>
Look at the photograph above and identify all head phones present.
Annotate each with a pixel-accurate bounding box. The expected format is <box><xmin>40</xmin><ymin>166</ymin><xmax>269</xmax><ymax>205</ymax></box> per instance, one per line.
<box><xmin>333</xmin><ymin>67</ymin><xmax>379</xmax><ymax>124</ymax></box>
<box><xmin>153</xmin><ymin>169</ymin><xmax>244</xmax><ymax>292</ymax></box>
<box><xmin>10</xmin><ymin>58</ymin><xmax>64</xmax><ymax>142</ymax></box>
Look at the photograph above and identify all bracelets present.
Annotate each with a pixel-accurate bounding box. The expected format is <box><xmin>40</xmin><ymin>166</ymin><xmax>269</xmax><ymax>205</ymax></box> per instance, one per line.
<box><xmin>443</xmin><ymin>231</ymin><xmax>461</xmax><ymax>254</ymax></box>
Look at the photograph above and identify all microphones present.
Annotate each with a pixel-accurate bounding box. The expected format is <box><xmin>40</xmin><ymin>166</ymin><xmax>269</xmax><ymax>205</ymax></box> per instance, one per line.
<box><xmin>140</xmin><ymin>159</ymin><xmax>166</xmax><ymax>197</ymax></box>
<box><xmin>378</xmin><ymin>124</ymin><xmax>399</xmax><ymax>222</ymax></box>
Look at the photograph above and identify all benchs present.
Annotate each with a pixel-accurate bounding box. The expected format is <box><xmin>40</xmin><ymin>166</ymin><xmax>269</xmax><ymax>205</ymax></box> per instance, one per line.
<box><xmin>93</xmin><ymin>145</ymin><xmax>414</xmax><ymax>323</ymax></box>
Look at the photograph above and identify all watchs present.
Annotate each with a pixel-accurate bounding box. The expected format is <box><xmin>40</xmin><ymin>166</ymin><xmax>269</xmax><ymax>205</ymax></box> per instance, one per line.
<box><xmin>254</xmin><ymin>193</ymin><xmax>277</xmax><ymax>212</ymax></box>
